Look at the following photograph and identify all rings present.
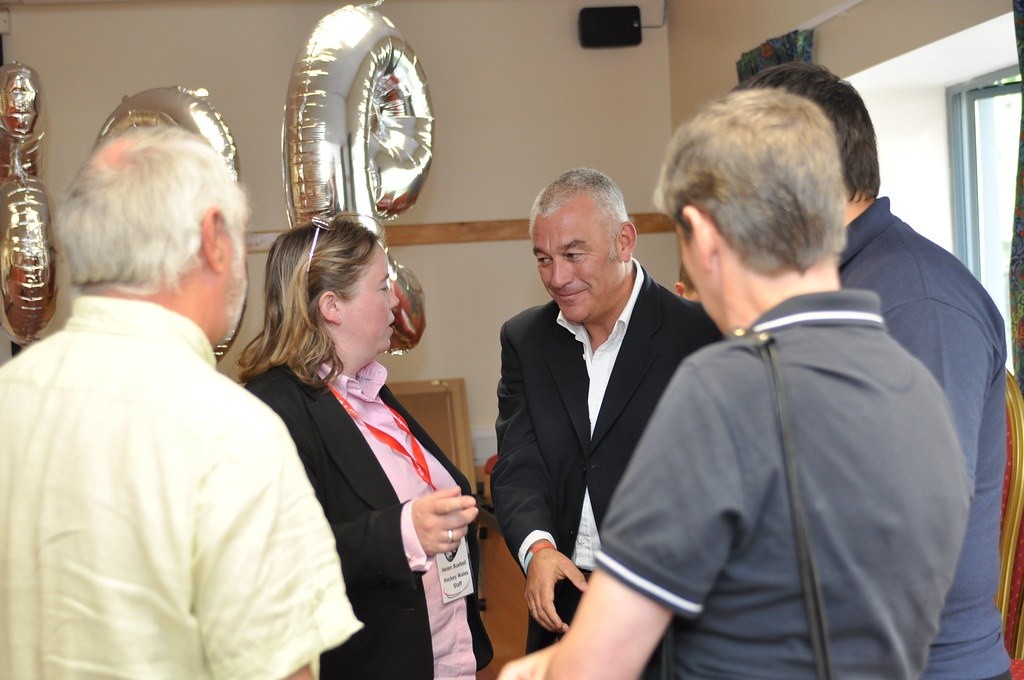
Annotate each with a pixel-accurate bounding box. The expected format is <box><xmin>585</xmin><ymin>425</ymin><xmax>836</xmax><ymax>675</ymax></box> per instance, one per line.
<box><xmin>448</xmin><ymin>530</ymin><xmax>453</xmax><ymax>543</ymax></box>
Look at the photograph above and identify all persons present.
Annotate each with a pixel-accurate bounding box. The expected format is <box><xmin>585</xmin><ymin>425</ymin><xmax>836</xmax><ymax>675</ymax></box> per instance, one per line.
<box><xmin>238</xmin><ymin>214</ymin><xmax>494</xmax><ymax>680</ymax></box>
<box><xmin>0</xmin><ymin>125</ymin><xmax>365</xmax><ymax>680</ymax></box>
<box><xmin>490</xmin><ymin>63</ymin><xmax>1011</xmax><ymax>679</ymax></box>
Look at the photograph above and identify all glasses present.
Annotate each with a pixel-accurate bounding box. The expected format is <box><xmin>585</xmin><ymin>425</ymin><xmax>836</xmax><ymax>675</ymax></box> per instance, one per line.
<box><xmin>306</xmin><ymin>216</ymin><xmax>336</xmax><ymax>273</ymax></box>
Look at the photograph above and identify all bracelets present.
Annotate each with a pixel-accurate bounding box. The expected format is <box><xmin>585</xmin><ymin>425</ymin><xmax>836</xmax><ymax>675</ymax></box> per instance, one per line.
<box><xmin>524</xmin><ymin>543</ymin><xmax>556</xmax><ymax>575</ymax></box>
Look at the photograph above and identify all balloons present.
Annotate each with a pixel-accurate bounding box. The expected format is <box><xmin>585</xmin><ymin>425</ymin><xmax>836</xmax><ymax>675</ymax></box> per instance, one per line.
<box><xmin>0</xmin><ymin>63</ymin><xmax>59</xmax><ymax>346</ymax></box>
<box><xmin>282</xmin><ymin>6</ymin><xmax>435</xmax><ymax>355</ymax></box>
